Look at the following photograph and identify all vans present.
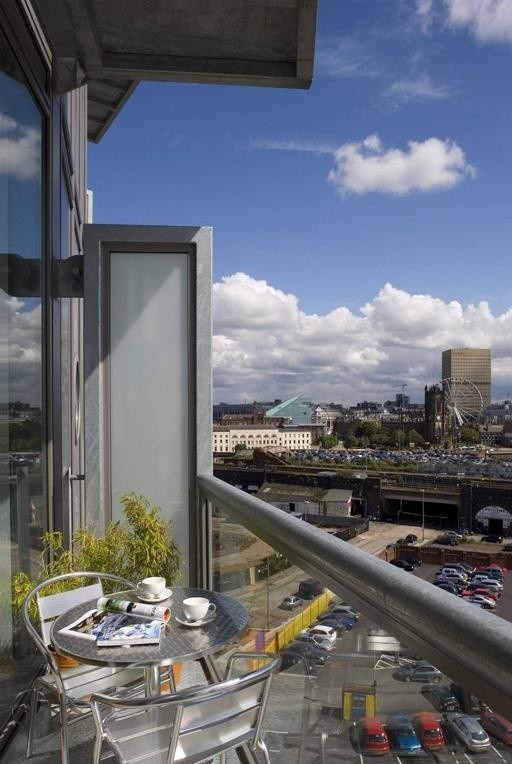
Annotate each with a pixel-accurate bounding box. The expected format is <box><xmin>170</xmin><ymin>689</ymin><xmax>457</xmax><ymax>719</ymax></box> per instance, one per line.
<box><xmin>298</xmin><ymin>578</ymin><xmax>324</xmax><ymax>599</ymax></box>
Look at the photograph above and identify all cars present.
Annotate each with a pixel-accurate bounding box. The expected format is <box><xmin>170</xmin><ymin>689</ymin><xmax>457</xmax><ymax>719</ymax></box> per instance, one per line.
<box><xmin>277</xmin><ymin>597</ymin><xmax>362</xmax><ymax>675</ymax></box>
<box><xmin>449</xmin><ymin>682</ymin><xmax>487</xmax><ymax>714</ymax></box>
<box><xmin>433</xmin><ymin>526</ymin><xmax>474</xmax><ymax>546</ymax></box>
<box><xmin>358</xmin><ymin>719</ymin><xmax>390</xmax><ymax>756</ymax></box>
<box><xmin>479</xmin><ymin>711</ymin><xmax>512</xmax><ymax>747</ymax></box>
<box><xmin>442</xmin><ymin>710</ymin><xmax>491</xmax><ymax>754</ymax></box>
<box><xmin>281</xmin><ymin>596</ymin><xmax>304</xmax><ymax>611</ymax></box>
<box><xmin>397</xmin><ymin>659</ymin><xmax>444</xmax><ymax>684</ymax></box>
<box><xmin>432</xmin><ymin>563</ymin><xmax>507</xmax><ymax>610</ymax></box>
<box><xmin>412</xmin><ymin>711</ymin><xmax>445</xmax><ymax>752</ymax></box>
<box><xmin>420</xmin><ymin>684</ymin><xmax>460</xmax><ymax>713</ymax></box>
<box><xmin>385</xmin><ymin>712</ymin><xmax>422</xmax><ymax>757</ymax></box>
<box><xmin>385</xmin><ymin>534</ymin><xmax>423</xmax><ymax>572</ymax></box>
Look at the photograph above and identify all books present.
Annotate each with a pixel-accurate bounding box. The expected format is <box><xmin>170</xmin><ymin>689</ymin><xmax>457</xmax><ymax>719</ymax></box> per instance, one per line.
<box><xmin>97</xmin><ymin>620</ymin><xmax>161</xmax><ymax>647</ymax></box>
<box><xmin>55</xmin><ymin>596</ymin><xmax>172</xmax><ymax>649</ymax></box>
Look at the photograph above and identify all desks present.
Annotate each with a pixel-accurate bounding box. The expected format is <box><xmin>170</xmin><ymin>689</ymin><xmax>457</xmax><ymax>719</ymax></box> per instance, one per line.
<box><xmin>48</xmin><ymin>585</ymin><xmax>248</xmax><ymax>714</ymax></box>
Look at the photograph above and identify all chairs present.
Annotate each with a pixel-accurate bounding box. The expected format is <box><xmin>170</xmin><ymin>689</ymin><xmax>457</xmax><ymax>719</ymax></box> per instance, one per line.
<box><xmin>87</xmin><ymin>650</ymin><xmax>282</xmax><ymax>764</ymax></box>
<box><xmin>23</xmin><ymin>570</ymin><xmax>177</xmax><ymax>764</ymax></box>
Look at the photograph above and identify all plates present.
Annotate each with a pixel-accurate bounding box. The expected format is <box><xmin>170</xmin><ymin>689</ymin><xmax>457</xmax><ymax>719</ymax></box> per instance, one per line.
<box><xmin>176</xmin><ymin>610</ymin><xmax>216</xmax><ymax>627</ymax></box>
<box><xmin>136</xmin><ymin>589</ymin><xmax>174</xmax><ymax>602</ymax></box>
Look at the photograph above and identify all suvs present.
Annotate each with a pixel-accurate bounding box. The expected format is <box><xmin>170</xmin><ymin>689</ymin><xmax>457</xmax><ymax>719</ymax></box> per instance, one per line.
<box><xmin>481</xmin><ymin>534</ymin><xmax>504</xmax><ymax>544</ymax></box>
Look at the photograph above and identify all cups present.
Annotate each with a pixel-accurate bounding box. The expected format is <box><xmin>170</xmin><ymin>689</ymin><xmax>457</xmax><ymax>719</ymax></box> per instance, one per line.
<box><xmin>183</xmin><ymin>597</ymin><xmax>214</xmax><ymax>621</ymax></box>
<box><xmin>137</xmin><ymin>577</ymin><xmax>166</xmax><ymax>596</ymax></box>
